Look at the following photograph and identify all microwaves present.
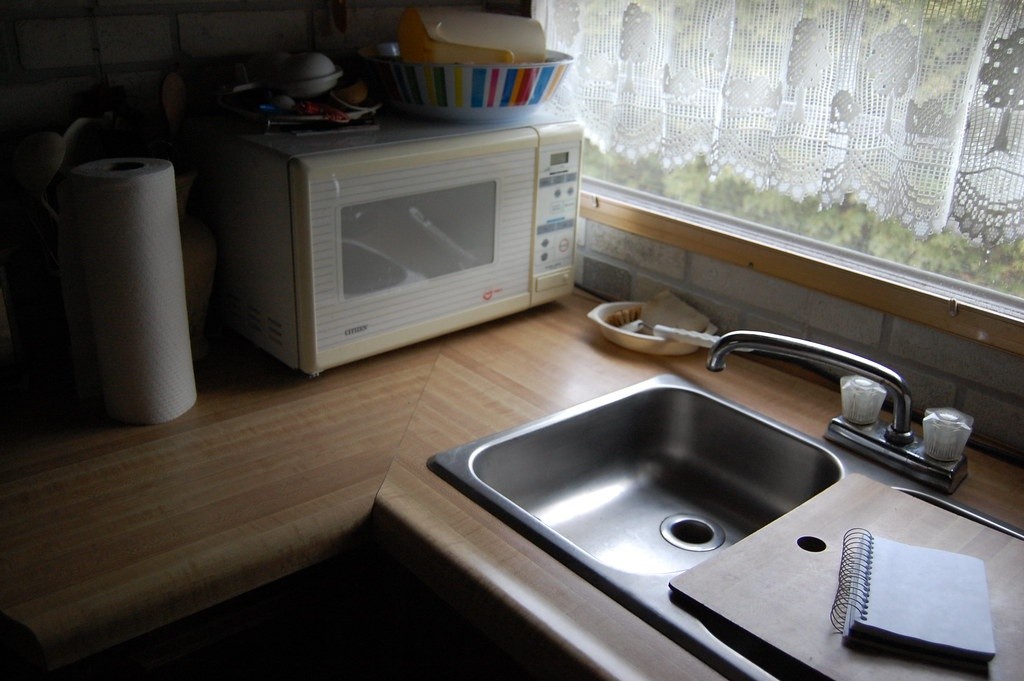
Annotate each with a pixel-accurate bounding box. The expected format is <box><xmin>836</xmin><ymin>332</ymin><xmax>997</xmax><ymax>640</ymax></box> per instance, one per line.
<box><xmin>210</xmin><ymin>109</ymin><xmax>581</xmax><ymax>377</ymax></box>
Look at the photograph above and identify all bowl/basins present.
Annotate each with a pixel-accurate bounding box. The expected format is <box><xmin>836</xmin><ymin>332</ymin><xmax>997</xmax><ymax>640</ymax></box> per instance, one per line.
<box><xmin>362</xmin><ymin>45</ymin><xmax>574</xmax><ymax>125</ymax></box>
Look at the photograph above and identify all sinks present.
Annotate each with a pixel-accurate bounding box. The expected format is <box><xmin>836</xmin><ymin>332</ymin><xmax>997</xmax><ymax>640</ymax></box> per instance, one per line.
<box><xmin>433</xmin><ymin>373</ymin><xmax>877</xmax><ymax>607</ymax></box>
<box><xmin>668</xmin><ymin>465</ymin><xmax>1024</xmax><ymax>681</ymax></box>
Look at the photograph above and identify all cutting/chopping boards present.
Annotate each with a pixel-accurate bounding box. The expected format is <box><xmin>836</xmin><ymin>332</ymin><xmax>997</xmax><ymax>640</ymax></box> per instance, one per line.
<box><xmin>667</xmin><ymin>472</ymin><xmax>1023</xmax><ymax>681</ymax></box>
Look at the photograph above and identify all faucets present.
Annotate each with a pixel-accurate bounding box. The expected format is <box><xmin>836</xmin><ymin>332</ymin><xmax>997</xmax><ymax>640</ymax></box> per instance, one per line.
<box><xmin>704</xmin><ymin>330</ymin><xmax>978</xmax><ymax>495</ymax></box>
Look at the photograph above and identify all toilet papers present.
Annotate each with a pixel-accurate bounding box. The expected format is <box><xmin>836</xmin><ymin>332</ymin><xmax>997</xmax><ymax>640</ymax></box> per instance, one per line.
<box><xmin>55</xmin><ymin>157</ymin><xmax>198</xmax><ymax>426</ymax></box>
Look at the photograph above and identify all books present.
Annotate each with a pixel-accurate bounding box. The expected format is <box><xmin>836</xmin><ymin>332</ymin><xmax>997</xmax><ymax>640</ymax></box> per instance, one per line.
<box><xmin>829</xmin><ymin>525</ymin><xmax>998</xmax><ymax>666</ymax></box>
